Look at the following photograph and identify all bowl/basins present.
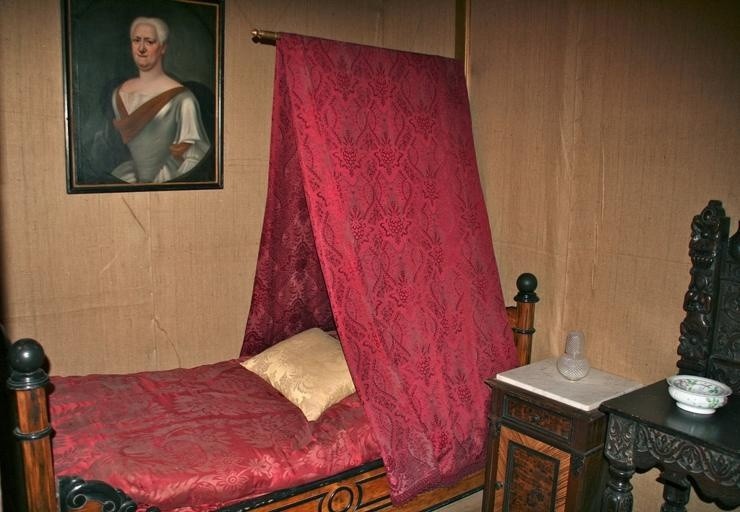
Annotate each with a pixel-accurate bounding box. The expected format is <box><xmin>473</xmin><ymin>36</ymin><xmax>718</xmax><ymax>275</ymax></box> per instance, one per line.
<box><xmin>664</xmin><ymin>373</ymin><xmax>734</xmax><ymax>414</ymax></box>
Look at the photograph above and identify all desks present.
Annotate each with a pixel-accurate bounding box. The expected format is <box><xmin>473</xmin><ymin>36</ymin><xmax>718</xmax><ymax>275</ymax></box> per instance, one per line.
<box><xmin>598</xmin><ymin>199</ymin><xmax>739</xmax><ymax>512</ymax></box>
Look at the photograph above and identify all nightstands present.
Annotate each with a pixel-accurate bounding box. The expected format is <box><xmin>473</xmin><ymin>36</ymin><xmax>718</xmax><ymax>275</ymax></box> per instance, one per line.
<box><xmin>483</xmin><ymin>355</ymin><xmax>643</xmax><ymax>512</ymax></box>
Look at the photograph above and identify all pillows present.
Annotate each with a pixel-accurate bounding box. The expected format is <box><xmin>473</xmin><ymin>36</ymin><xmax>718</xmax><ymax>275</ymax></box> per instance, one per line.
<box><xmin>238</xmin><ymin>328</ymin><xmax>355</xmax><ymax>423</ymax></box>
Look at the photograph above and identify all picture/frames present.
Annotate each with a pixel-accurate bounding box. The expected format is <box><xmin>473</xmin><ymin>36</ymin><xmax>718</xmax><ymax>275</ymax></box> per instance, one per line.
<box><xmin>61</xmin><ymin>1</ymin><xmax>222</xmax><ymax>193</ymax></box>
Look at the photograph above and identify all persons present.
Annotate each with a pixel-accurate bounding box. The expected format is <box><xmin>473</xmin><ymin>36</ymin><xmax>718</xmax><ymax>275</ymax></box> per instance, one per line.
<box><xmin>73</xmin><ymin>13</ymin><xmax>212</xmax><ymax>184</ymax></box>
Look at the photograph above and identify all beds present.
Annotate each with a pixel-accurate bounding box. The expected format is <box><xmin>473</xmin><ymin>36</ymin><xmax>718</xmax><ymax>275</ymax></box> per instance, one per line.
<box><xmin>0</xmin><ymin>30</ymin><xmax>539</xmax><ymax>512</ymax></box>
